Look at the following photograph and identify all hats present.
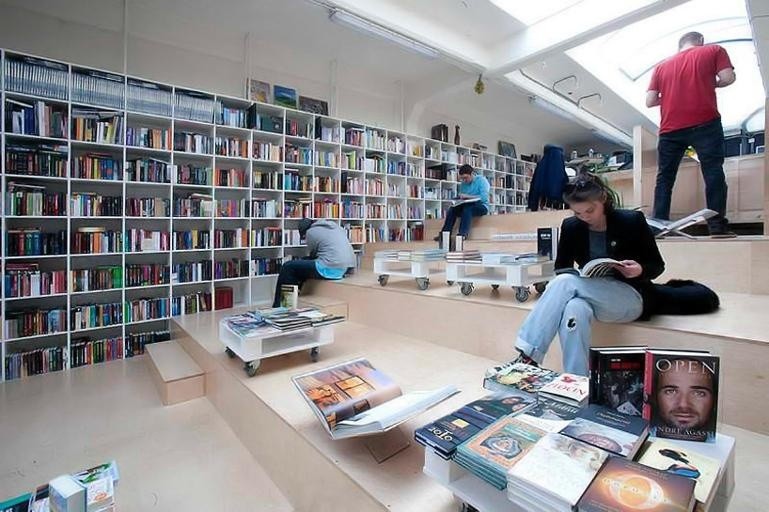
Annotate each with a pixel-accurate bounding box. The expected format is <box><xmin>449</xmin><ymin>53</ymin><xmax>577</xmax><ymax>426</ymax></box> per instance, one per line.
<box><xmin>298</xmin><ymin>218</ymin><xmax>313</xmax><ymax>239</ymax></box>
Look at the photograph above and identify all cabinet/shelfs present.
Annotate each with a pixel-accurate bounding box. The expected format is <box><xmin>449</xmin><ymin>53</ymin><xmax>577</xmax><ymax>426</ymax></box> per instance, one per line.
<box><xmin>0</xmin><ymin>48</ymin><xmax>539</xmax><ymax>382</ymax></box>
<box><xmin>444</xmin><ymin>261</ymin><xmax>555</xmax><ymax>301</ymax></box>
<box><xmin>373</xmin><ymin>257</ymin><xmax>445</xmax><ymax>290</ymax></box>
<box><xmin>218</xmin><ymin>306</ymin><xmax>335</xmax><ymax>376</ymax></box>
<box><xmin>421</xmin><ymin>433</ymin><xmax>736</xmax><ymax>511</ymax></box>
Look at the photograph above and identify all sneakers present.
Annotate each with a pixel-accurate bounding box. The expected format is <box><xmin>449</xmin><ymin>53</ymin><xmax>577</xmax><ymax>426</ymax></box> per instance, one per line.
<box><xmin>485</xmin><ymin>353</ymin><xmax>539</xmax><ymax>379</ymax></box>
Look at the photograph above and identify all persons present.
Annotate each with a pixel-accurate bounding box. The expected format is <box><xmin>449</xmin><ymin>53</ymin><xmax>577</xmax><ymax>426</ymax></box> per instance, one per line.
<box><xmin>434</xmin><ymin>164</ymin><xmax>490</xmax><ymax>239</ymax></box>
<box><xmin>486</xmin><ymin>162</ymin><xmax>718</xmax><ymax>374</ymax></box>
<box><xmin>271</xmin><ymin>217</ymin><xmax>356</xmax><ymax>308</ymax></box>
<box><xmin>645</xmin><ymin>32</ymin><xmax>737</xmax><ymax>235</ymax></box>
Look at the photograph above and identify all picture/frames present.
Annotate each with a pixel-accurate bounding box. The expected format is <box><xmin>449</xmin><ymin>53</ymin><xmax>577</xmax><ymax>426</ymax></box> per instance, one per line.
<box><xmin>271</xmin><ymin>83</ymin><xmax>299</xmax><ymax>110</ymax></box>
<box><xmin>248</xmin><ymin>77</ymin><xmax>272</xmax><ymax>103</ymax></box>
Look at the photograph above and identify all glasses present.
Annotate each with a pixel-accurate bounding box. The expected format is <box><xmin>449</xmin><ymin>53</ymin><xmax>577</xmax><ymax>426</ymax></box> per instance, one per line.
<box><xmin>565</xmin><ymin>181</ymin><xmax>592</xmax><ymax>194</ymax></box>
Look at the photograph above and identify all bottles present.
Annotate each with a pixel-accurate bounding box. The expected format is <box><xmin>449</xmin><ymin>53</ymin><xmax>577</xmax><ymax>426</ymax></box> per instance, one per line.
<box><xmin>572</xmin><ymin>151</ymin><xmax>577</xmax><ymax>159</ymax></box>
<box><xmin>454</xmin><ymin>125</ymin><xmax>461</xmax><ymax>145</ymax></box>
<box><xmin>588</xmin><ymin>148</ymin><xmax>594</xmax><ymax>158</ymax></box>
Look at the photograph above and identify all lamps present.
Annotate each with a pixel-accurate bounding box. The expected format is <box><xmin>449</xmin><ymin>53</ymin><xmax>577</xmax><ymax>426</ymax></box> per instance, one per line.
<box><xmin>506</xmin><ymin>68</ymin><xmax>634</xmax><ymax>155</ymax></box>
<box><xmin>326</xmin><ymin>10</ymin><xmax>439</xmax><ymax>60</ymax></box>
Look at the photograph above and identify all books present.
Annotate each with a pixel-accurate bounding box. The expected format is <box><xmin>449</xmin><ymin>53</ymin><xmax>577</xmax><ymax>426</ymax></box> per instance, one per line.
<box><xmin>1</xmin><ymin>460</ymin><xmax>119</xmax><ymax>512</ymax></box>
<box><xmin>415</xmin><ymin>344</ymin><xmax>719</xmax><ymax>512</ymax></box>
<box><xmin>215</xmin><ymin>97</ymin><xmax>257</xmax><ymax>311</ymax></box>
<box><xmin>292</xmin><ymin>356</ymin><xmax>462</xmax><ymax>441</ymax></box>
<box><xmin>425</xmin><ymin>143</ymin><xmax>483</xmax><ymax>221</ymax></box>
<box><xmin>172</xmin><ymin>87</ymin><xmax>214</xmax><ymax>315</ymax></box>
<box><xmin>484</xmin><ymin>157</ymin><xmax>532</xmax><ymax>216</ymax></box>
<box><xmin>365</xmin><ymin>127</ymin><xmax>385</xmax><ymax>243</ymax></box>
<box><xmin>284</xmin><ymin>118</ymin><xmax>314</xmax><ymax>265</ymax></box>
<box><xmin>124</xmin><ymin>77</ymin><xmax>173</xmax><ymax>360</ymax></box>
<box><xmin>340</xmin><ymin>127</ymin><xmax>364</xmax><ymax>272</ymax></box>
<box><xmin>4</xmin><ymin>51</ymin><xmax>67</xmax><ymax>380</ymax></box>
<box><xmin>406</xmin><ymin>141</ymin><xmax>424</xmax><ymax>242</ymax></box>
<box><xmin>315</xmin><ymin>117</ymin><xmax>339</xmax><ymax>217</ymax></box>
<box><xmin>252</xmin><ymin>109</ymin><xmax>283</xmax><ymax>276</ymax></box>
<box><xmin>226</xmin><ymin>285</ymin><xmax>345</xmax><ymax>335</ymax></box>
<box><xmin>568</xmin><ymin>255</ymin><xmax>625</xmax><ymax>278</ymax></box>
<box><xmin>387</xmin><ymin>135</ymin><xmax>405</xmax><ymax>242</ymax></box>
<box><xmin>69</xmin><ymin>63</ymin><xmax>124</xmax><ymax>368</ymax></box>
<box><xmin>516</xmin><ymin>226</ymin><xmax>557</xmax><ymax>262</ymax></box>
<box><xmin>646</xmin><ymin>207</ymin><xmax>719</xmax><ymax>230</ymax></box>
